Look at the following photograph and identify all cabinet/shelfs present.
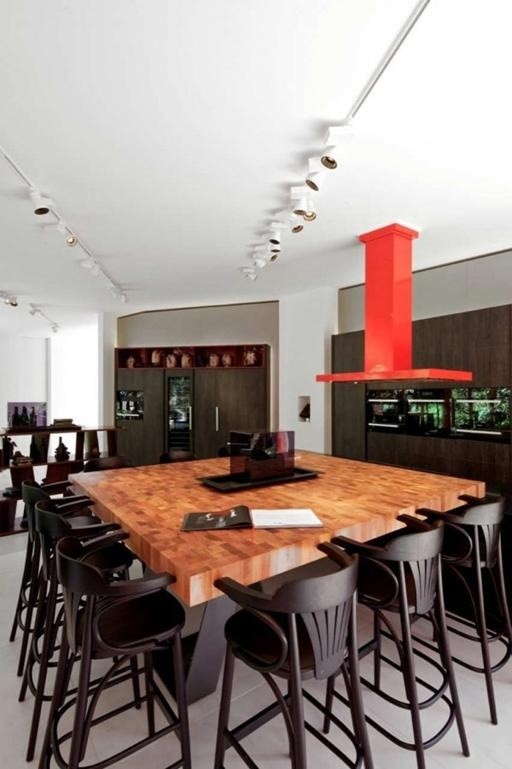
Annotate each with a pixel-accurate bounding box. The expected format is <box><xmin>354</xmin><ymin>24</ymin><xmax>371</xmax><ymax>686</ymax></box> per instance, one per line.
<box><xmin>114</xmin><ymin>346</ymin><xmax>269</xmax><ymax>465</ymax></box>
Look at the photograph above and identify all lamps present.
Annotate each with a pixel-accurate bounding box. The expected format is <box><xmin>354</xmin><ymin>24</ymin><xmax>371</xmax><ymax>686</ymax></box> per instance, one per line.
<box><xmin>30</xmin><ymin>193</ymin><xmax>78</xmax><ymax>249</ymax></box>
<box><xmin>235</xmin><ymin>125</ymin><xmax>349</xmax><ymax>281</ymax></box>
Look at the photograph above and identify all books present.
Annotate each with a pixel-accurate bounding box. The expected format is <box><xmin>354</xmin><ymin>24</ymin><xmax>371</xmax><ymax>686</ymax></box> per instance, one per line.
<box><xmin>181</xmin><ymin>505</ymin><xmax>324</xmax><ymax>531</ymax></box>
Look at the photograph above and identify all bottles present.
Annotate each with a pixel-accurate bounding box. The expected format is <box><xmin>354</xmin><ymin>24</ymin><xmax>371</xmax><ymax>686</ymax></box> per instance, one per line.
<box><xmin>11</xmin><ymin>405</ymin><xmax>37</xmax><ymax>429</ymax></box>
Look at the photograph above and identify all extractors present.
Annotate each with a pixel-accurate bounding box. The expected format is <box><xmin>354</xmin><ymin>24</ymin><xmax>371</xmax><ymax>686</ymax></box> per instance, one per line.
<box><xmin>314</xmin><ymin>222</ymin><xmax>473</xmax><ymax>383</ymax></box>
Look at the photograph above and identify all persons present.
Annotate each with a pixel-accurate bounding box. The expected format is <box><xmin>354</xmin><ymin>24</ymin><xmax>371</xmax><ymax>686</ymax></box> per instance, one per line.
<box><xmin>116</xmin><ymin>391</ymin><xmax>143</xmax><ymax>414</ymax></box>
<box><xmin>20</xmin><ymin>406</ymin><xmax>29</xmax><ymax>425</ymax></box>
<box><xmin>12</xmin><ymin>406</ymin><xmax>20</xmax><ymax>426</ymax></box>
<box><xmin>30</xmin><ymin>406</ymin><xmax>36</xmax><ymax>427</ymax></box>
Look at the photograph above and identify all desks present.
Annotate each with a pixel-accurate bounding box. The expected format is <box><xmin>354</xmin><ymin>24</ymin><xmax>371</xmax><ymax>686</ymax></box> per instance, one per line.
<box><xmin>69</xmin><ymin>450</ymin><xmax>486</xmax><ymax>708</ymax></box>
